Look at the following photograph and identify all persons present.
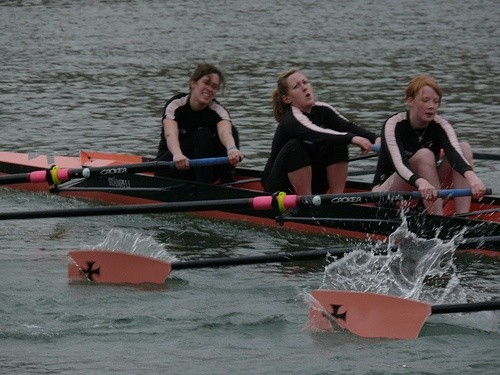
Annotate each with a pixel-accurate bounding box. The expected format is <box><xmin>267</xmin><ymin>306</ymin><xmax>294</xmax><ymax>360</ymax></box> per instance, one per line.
<box><xmin>371</xmin><ymin>76</ymin><xmax>486</xmax><ymax>219</ymax></box>
<box><xmin>154</xmin><ymin>64</ymin><xmax>243</xmax><ymax>186</ymax></box>
<box><xmin>261</xmin><ymin>69</ymin><xmax>380</xmax><ymax>201</ymax></box>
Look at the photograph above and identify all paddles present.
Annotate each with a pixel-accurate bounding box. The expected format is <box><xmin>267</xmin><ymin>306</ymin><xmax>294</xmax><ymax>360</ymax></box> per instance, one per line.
<box><xmin>0</xmin><ymin>188</ymin><xmax>493</xmax><ymax>219</ymax></box>
<box><xmin>0</xmin><ymin>156</ymin><xmax>242</xmax><ymax>184</ymax></box>
<box><xmin>304</xmin><ymin>289</ymin><xmax>500</xmax><ymax>339</ymax></box>
<box><xmin>65</xmin><ymin>236</ymin><xmax>500</xmax><ymax>287</ymax></box>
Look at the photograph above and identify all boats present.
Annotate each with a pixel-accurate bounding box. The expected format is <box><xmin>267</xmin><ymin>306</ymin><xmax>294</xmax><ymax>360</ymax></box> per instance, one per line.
<box><xmin>0</xmin><ymin>150</ymin><xmax>500</xmax><ymax>257</ymax></box>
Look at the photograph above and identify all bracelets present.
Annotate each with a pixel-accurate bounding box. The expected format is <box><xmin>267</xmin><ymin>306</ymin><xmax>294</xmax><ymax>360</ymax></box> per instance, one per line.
<box><xmin>227</xmin><ymin>146</ymin><xmax>237</xmax><ymax>151</ymax></box>
